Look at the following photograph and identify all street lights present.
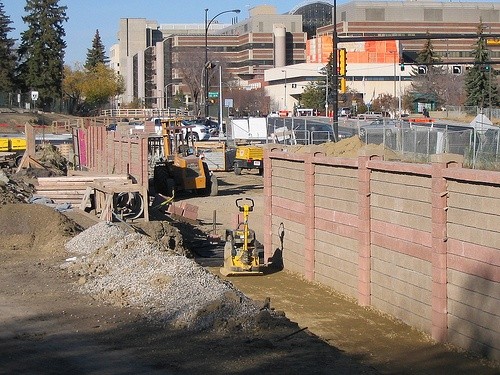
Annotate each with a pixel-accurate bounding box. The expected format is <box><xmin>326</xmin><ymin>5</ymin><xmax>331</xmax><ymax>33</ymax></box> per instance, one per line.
<box><xmin>203</xmin><ymin>7</ymin><xmax>241</xmax><ymax>118</ymax></box>
<box><xmin>281</xmin><ymin>69</ymin><xmax>287</xmax><ymax>108</ymax></box>
<box><xmin>480</xmin><ymin>66</ymin><xmax>489</xmax><ymax>71</ymax></box>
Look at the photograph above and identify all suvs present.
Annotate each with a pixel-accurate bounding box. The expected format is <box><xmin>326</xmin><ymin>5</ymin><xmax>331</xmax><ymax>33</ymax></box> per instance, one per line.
<box><xmin>337</xmin><ymin>107</ymin><xmax>351</xmax><ymax>117</ymax></box>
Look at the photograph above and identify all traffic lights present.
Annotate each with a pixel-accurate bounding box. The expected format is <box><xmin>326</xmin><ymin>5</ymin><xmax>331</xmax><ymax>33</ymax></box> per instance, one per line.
<box><xmin>401</xmin><ymin>59</ymin><xmax>404</xmax><ymax>71</ymax></box>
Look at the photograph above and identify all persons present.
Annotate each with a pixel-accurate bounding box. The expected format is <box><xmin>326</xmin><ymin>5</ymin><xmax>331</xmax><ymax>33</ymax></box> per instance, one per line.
<box><xmin>205</xmin><ymin>119</ymin><xmax>211</xmax><ymax>127</ymax></box>
<box><xmin>423</xmin><ymin>107</ymin><xmax>430</xmax><ymax>118</ymax></box>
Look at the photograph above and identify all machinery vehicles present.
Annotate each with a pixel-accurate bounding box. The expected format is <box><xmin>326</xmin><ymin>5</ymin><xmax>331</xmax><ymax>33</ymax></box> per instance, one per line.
<box><xmin>149</xmin><ymin>120</ymin><xmax>218</xmax><ymax>197</ymax></box>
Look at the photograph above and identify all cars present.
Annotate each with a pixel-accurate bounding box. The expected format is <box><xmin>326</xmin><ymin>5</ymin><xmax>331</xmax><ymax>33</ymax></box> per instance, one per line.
<box><xmin>359</xmin><ymin>119</ymin><xmax>411</xmax><ymax>139</ymax></box>
<box><xmin>358</xmin><ymin>111</ymin><xmax>383</xmax><ymax>120</ymax></box>
<box><xmin>107</xmin><ymin>114</ymin><xmax>226</xmax><ymax>142</ymax></box>
